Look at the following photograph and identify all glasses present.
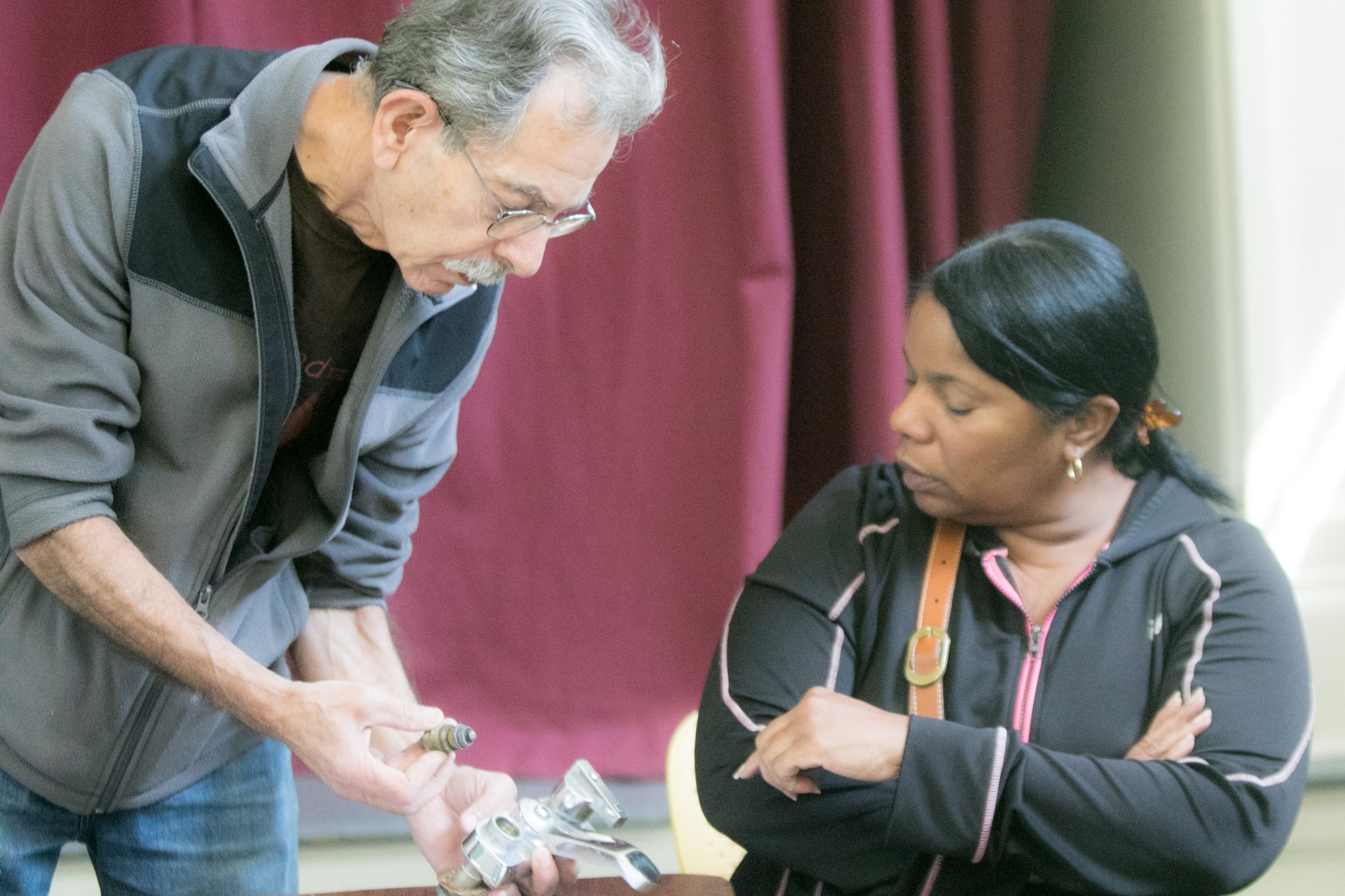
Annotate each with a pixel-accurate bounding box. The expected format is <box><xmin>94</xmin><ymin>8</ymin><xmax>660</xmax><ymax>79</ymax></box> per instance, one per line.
<box><xmin>404</xmin><ymin>82</ymin><xmax>595</xmax><ymax>244</ymax></box>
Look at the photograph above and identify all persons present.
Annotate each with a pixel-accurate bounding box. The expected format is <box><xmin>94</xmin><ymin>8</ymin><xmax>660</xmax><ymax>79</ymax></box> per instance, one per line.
<box><xmin>696</xmin><ymin>218</ymin><xmax>1312</xmax><ymax>896</ymax></box>
<box><xmin>0</xmin><ymin>0</ymin><xmax>674</xmax><ymax>896</ymax></box>
